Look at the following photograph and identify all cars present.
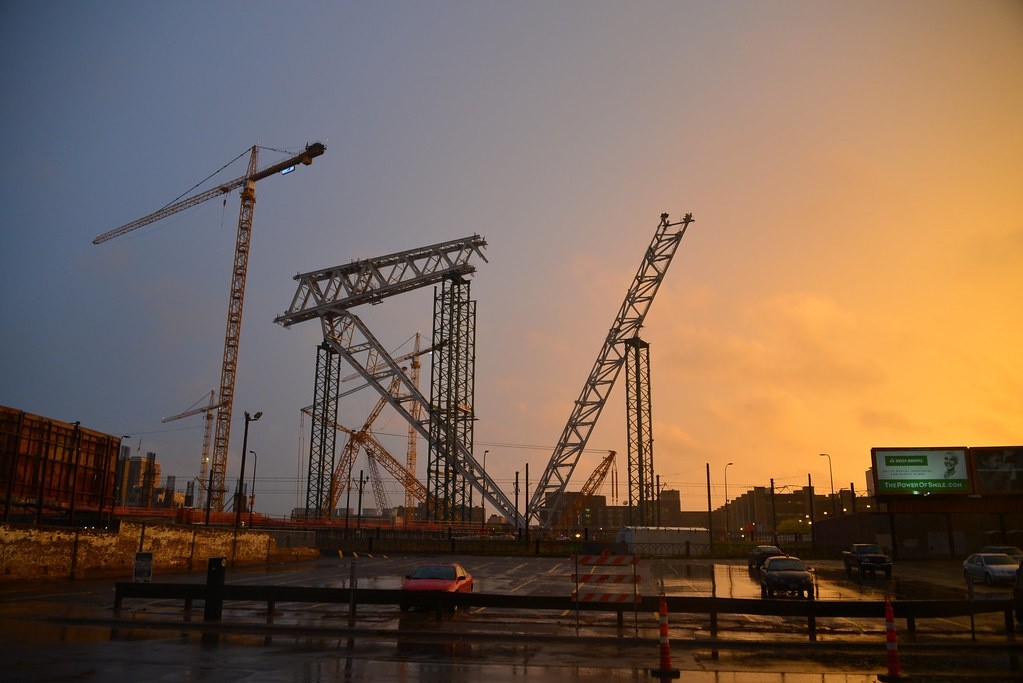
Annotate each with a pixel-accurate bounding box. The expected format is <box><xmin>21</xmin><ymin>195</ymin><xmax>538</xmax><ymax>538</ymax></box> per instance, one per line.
<box><xmin>975</xmin><ymin>545</ymin><xmax>1023</xmax><ymax>565</ymax></box>
<box><xmin>962</xmin><ymin>552</ymin><xmax>1020</xmax><ymax>587</ymax></box>
<box><xmin>758</xmin><ymin>555</ymin><xmax>814</xmax><ymax>600</ymax></box>
<box><xmin>398</xmin><ymin>561</ymin><xmax>474</xmax><ymax>616</ymax></box>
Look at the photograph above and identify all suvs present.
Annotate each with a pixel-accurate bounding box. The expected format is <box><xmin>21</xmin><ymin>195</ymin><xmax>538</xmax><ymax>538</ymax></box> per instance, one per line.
<box><xmin>747</xmin><ymin>544</ymin><xmax>785</xmax><ymax>580</ymax></box>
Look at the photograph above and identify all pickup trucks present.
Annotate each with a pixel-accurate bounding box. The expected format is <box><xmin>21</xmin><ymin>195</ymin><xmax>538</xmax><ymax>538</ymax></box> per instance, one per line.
<box><xmin>843</xmin><ymin>543</ymin><xmax>893</xmax><ymax>580</ymax></box>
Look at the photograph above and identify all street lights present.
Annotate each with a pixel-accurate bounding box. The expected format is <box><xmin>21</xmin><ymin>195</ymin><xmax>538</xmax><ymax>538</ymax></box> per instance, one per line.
<box><xmin>106</xmin><ymin>435</ymin><xmax>131</xmax><ymax>533</ymax></box>
<box><xmin>249</xmin><ymin>451</ymin><xmax>258</xmax><ymax>529</ymax></box>
<box><xmin>724</xmin><ymin>462</ymin><xmax>733</xmax><ymax>540</ymax></box>
<box><xmin>482</xmin><ymin>450</ymin><xmax>490</xmax><ymax>533</ymax></box>
<box><xmin>344</xmin><ymin>429</ymin><xmax>357</xmax><ymax>535</ymax></box>
<box><xmin>820</xmin><ymin>453</ymin><xmax>836</xmax><ymax>518</ymax></box>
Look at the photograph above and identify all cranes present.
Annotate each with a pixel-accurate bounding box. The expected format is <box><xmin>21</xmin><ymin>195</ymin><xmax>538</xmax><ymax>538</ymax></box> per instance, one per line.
<box><xmin>288</xmin><ymin>330</ymin><xmax>480</xmax><ymax>534</ymax></box>
<box><xmin>90</xmin><ymin>141</ymin><xmax>330</xmax><ymax>514</ymax></box>
<box><xmin>161</xmin><ymin>389</ymin><xmax>232</xmax><ymax>505</ymax></box>
